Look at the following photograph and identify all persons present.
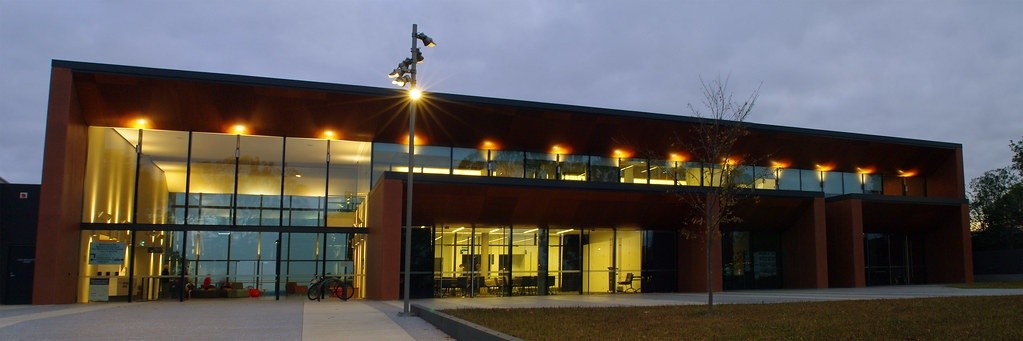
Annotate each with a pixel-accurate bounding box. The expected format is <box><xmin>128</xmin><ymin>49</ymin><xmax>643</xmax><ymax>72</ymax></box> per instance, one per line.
<box><xmin>203</xmin><ymin>274</ymin><xmax>211</xmax><ymax>289</ymax></box>
<box><xmin>186</xmin><ymin>281</ymin><xmax>194</xmax><ymax>296</ymax></box>
<box><xmin>162</xmin><ymin>265</ymin><xmax>170</xmax><ymax>290</ymax></box>
<box><xmin>184</xmin><ymin>266</ymin><xmax>190</xmax><ymax>275</ymax></box>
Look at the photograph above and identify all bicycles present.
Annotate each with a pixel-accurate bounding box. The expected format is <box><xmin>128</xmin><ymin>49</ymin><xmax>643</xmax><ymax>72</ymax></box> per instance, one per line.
<box><xmin>307</xmin><ymin>274</ymin><xmax>354</xmax><ymax>300</ymax></box>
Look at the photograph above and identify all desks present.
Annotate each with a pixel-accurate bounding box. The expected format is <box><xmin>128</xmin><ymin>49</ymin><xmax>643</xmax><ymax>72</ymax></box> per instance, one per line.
<box><xmin>435</xmin><ymin>278</ymin><xmax>476</xmax><ymax>297</ymax></box>
<box><xmin>141</xmin><ymin>276</ymin><xmax>190</xmax><ymax>301</ymax></box>
<box><xmin>514</xmin><ymin>276</ymin><xmax>557</xmax><ymax>295</ymax></box>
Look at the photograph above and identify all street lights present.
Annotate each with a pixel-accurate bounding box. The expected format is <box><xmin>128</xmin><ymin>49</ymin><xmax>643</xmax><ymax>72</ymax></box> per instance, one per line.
<box><xmin>388</xmin><ymin>24</ymin><xmax>436</xmax><ymax>317</ymax></box>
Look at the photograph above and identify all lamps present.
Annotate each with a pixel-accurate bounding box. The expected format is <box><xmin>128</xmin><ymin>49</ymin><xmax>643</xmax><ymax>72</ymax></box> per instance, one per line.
<box><xmin>109</xmin><ymin>231</ymin><xmax>113</xmax><ymax>240</ymax></box>
<box><xmin>116</xmin><ymin>232</ymin><xmax>120</xmax><ymax>243</ymax></box>
<box><xmin>93</xmin><ymin>230</ymin><xmax>99</xmax><ymax>237</ymax></box>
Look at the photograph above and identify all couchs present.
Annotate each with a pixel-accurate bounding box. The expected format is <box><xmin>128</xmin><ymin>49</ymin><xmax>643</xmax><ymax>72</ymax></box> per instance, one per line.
<box><xmin>287</xmin><ymin>282</ymin><xmax>308</xmax><ymax>295</ymax></box>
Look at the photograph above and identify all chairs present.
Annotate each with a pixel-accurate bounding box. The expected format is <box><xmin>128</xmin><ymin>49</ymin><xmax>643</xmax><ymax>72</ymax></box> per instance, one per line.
<box><xmin>494</xmin><ymin>278</ymin><xmax>502</xmax><ymax>295</ymax></box>
<box><xmin>520</xmin><ymin>276</ymin><xmax>533</xmax><ymax>296</ymax></box>
<box><xmin>502</xmin><ymin>277</ymin><xmax>508</xmax><ymax>297</ymax></box>
<box><xmin>534</xmin><ymin>276</ymin><xmax>538</xmax><ymax>295</ymax></box>
<box><xmin>617</xmin><ymin>273</ymin><xmax>638</xmax><ymax>294</ymax></box>
<box><xmin>475</xmin><ymin>276</ymin><xmax>487</xmax><ymax>296</ymax></box>
<box><xmin>451</xmin><ymin>276</ymin><xmax>468</xmax><ymax>297</ymax></box>
